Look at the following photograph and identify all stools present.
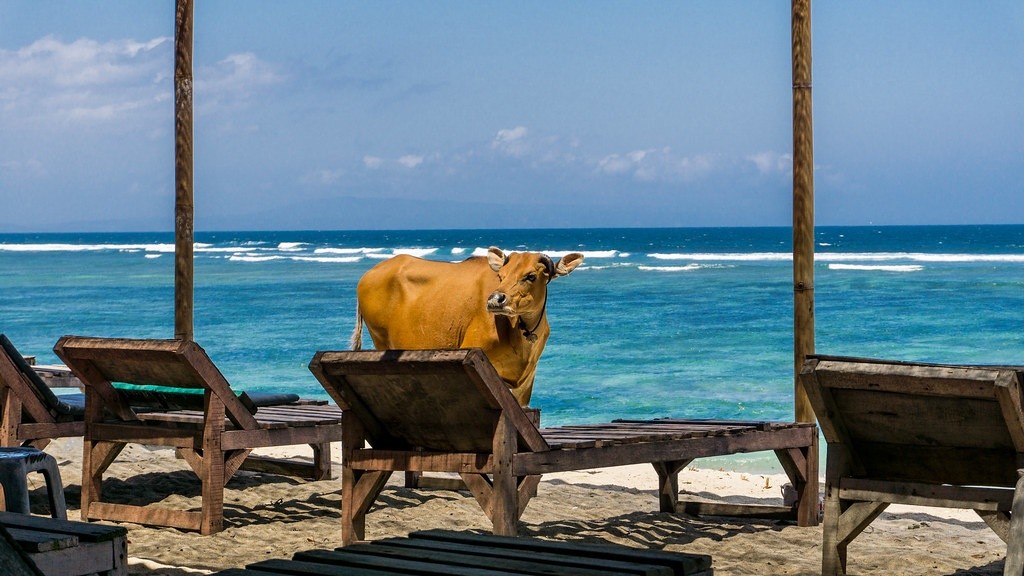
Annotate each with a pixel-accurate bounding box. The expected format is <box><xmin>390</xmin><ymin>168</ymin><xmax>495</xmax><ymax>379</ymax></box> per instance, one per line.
<box><xmin>0</xmin><ymin>446</ymin><xmax>68</xmax><ymax>521</ymax></box>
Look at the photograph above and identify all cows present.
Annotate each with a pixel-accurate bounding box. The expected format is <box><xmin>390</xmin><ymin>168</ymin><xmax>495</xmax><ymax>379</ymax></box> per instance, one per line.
<box><xmin>349</xmin><ymin>245</ymin><xmax>584</xmax><ymax>407</ymax></box>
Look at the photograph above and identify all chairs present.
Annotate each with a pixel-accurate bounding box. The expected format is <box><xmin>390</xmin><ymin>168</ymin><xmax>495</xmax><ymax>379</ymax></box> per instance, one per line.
<box><xmin>205</xmin><ymin>527</ymin><xmax>713</xmax><ymax>576</ymax></box>
<box><xmin>0</xmin><ymin>333</ymin><xmax>85</xmax><ymax>514</ymax></box>
<box><xmin>54</xmin><ymin>335</ymin><xmax>343</xmax><ymax>534</ymax></box>
<box><xmin>799</xmin><ymin>353</ymin><xmax>1024</xmax><ymax>576</ymax></box>
<box><xmin>0</xmin><ymin>511</ymin><xmax>128</xmax><ymax>576</ymax></box>
<box><xmin>309</xmin><ymin>350</ymin><xmax>819</xmax><ymax>545</ymax></box>
